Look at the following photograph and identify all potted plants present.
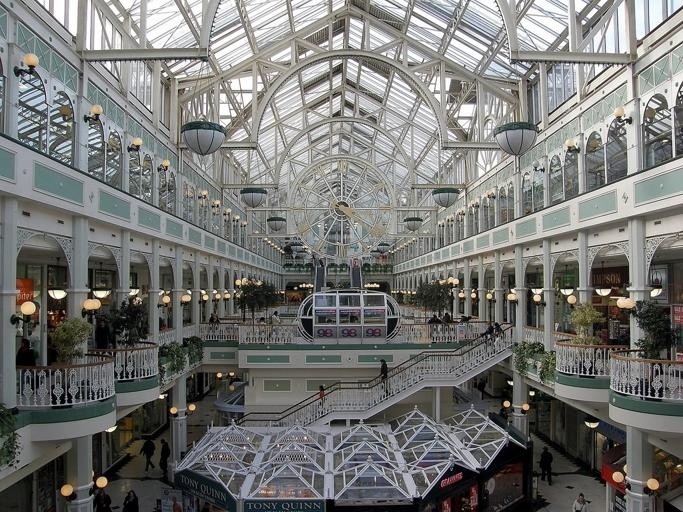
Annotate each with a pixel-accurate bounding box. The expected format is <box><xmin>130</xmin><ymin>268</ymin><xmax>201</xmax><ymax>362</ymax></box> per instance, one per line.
<box><xmin>101</xmin><ymin>298</ymin><xmax>148</xmax><ymax>377</ymax></box>
<box><xmin>47</xmin><ymin>317</ymin><xmax>91</xmax><ymax>400</ymax></box>
<box><xmin>563</xmin><ymin>299</ymin><xmax>604</xmax><ymax>375</ymax></box>
<box><xmin>628</xmin><ymin>295</ymin><xmax>683</xmax><ymax>401</ymax></box>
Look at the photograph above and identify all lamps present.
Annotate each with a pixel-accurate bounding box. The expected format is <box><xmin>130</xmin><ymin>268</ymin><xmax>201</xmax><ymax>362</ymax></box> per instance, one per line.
<box><xmin>489</xmin><ymin>375</ymin><xmax>672</xmax><ymax>509</ymax></box>
<box><xmin>617</xmin><ymin>298</ymin><xmax>628</xmax><ymax>309</ymax></box>
<box><xmin>13</xmin><ymin>301</ymin><xmax>36</xmax><ymax>322</ymax></box>
<box><xmin>533</xmin><ymin>295</ymin><xmax>545</xmax><ymax>306</ymax></box>
<box><xmin>567</xmin><ymin>295</ymin><xmax>576</xmax><ymax>310</ymax></box>
<box><xmin>128</xmin><ymin>267</ymin><xmax>140</xmax><ymax>296</ymax></box>
<box><xmin>181</xmin><ymin>58</ymin><xmax>226</xmax><ymax>156</ymax></box>
<box><xmin>92</xmin><ymin>262</ymin><xmax>112</xmax><ymax>300</ymax></box>
<box><xmin>596</xmin><ymin>261</ymin><xmax>611</xmax><ymax>296</ymax></box>
<box><xmin>180</xmin><ymin>272</ymin><xmax>193</xmax><ymax>306</ymax></box>
<box><xmin>560</xmin><ymin>265</ymin><xmax>574</xmax><ymax>295</ymax></box>
<box><xmin>403</xmin><ymin>188</ymin><xmax>423</xmax><ymax>232</ymax></box>
<box><xmin>159</xmin><ymin>271</ymin><xmax>171</xmax><ymax>308</ymax></box>
<box><xmin>84</xmin><ymin>104</ymin><xmax>103</xmax><ymax>123</ymax></box>
<box><xmin>14</xmin><ymin>53</ymin><xmax>39</xmax><ymax>77</ymax></box>
<box><xmin>431</xmin><ymin>148</ymin><xmax>461</xmax><ymax>208</ymax></box>
<box><xmin>493</xmin><ymin>63</ymin><xmax>540</xmax><ymax>156</ymax></box>
<box><xmin>267</xmin><ymin>188</ymin><xmax>287</xmax><ymax>231</ymax></box>
<box><xmin>52</xmin><ymin>370</ymin><xmax>236</xmax><ymax>508</ymax></box>
<box><xmin>240</xmin><ymin>148</ymin><xmax>268</xmax><ymax>208</ymax></box>
<box><xmin>129</xmin><ymin>138</ymin><xmax>142</xmax><ymax>151</ymax></box>
<box><xmin>82</xmin><ymin>299</ymin><xmax>102</xmax><ymax>316</ymax></box>
<box><xmin>48</xmin><ymin>256</ymin><xmax>67</xmax><ymax>299</ymax></box>
<box><xmin>157</xmin><ymin>159</ymin><xmax>171</xmax><ymax>173</ymax></box>
<box><xmin>200</xmin><ymin>289</ymin><xmax>243</xmax><ymax>304</ymax></box>
<box><xmin>531</xmin><ymin>268</ymin><xmax>542</xmax><ymax>294</ymax></box>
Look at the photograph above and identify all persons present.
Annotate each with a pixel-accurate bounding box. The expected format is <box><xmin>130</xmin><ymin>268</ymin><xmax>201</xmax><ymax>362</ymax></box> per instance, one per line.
<box><xmin>159</xmin><ymin>438</ymin><xmax>170</xmax><ymax>474</ymax></box>
<box><xmin>427</xmin><ymin>313</ymin><xmax>503</xmax><ymax>353</ymax></box>
<box><xmin>121</xmin><ymin>489</ymin><xmax>139</xmax><ymax>512</ymax></box>
<box><xmin>380</xmin><ymin>359</ymin><xmax>389</xmax><ymax>396</ymax></box>
<box><xmin>538</xmin><ymin>446</ymin><xmax>552</xmax><ymax>486</ymax></box>
<box><xmin>478</xmin><ymin>378</ymin><xmax>486</xmax><ymax>401</ymax></box>
<box><xmin>138</xmin><ymin>438</ymin><xmax>155</xmax><ymax>474</ymax></box>
<box><xmin>271</xmin><ymin>310</ymin><xmax>280</xmax><ymax>344</ymax></box>
<box><xmin>209</xmin><ymin>313</ymin><xmax>218</xmax><ymax>342</ymax></box>
<box><xmin>16</xmin><ymin>339</ymin><xmax>36</xmax><ymax>394</ymax></box>
<box><xmin>257</xmin><ymin>316</ymin><xmax>267</xmax><ymax>343</ymax></box>
<box><xmin>92</xmin><ymin>488</ymin><xmax>112</xmax><ymax>512</ymax></box>
<box><xmin>317</xmin><ymin>385</ymin><xmax>325</xmax><ymax>414</ymax></box>
<box><xmin>571</xmin><ymin>493</ymin><xmax>586</xmax><ymax>512</ymax></box>
